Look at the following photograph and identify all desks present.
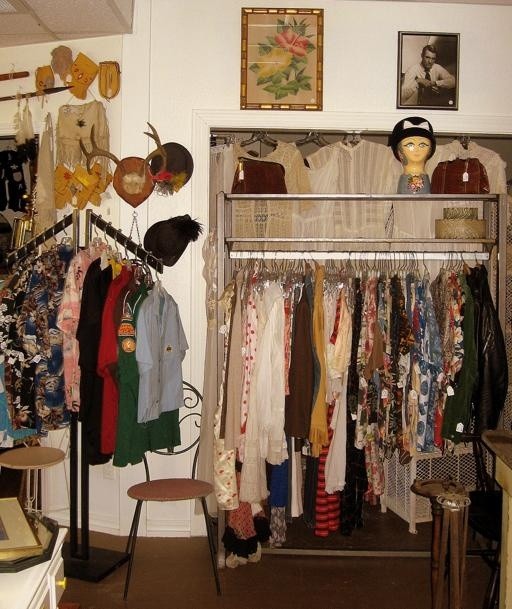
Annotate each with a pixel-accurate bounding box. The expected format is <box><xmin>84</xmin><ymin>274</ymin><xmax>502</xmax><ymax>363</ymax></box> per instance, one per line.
<box><xmin>480</xmin><ymin>430</ymin><xmax>512</xmax><ymax>609</ymax></box>
<box><xmin>0</xmin><ymin>527</ymin><xmax>68</xmax><ymax>609</ymax></box>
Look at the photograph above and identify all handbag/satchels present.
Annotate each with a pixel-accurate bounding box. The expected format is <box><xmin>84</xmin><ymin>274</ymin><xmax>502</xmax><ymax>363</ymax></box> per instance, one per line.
<box><xmin>231</xmin><ymin>157</ymin><xmax>288</xmax><ymax>194</ymax></box>
<box><xmin>430</xmin><ymin>156</ymin><xmax>490</xmax><ymax>194</ymax></box>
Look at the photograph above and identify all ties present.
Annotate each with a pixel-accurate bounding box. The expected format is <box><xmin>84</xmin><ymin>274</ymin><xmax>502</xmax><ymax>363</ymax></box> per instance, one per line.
<box><xmin>425</xmin><ymin>70</ymin><xmax>432</xmax><ymax>96</ymax></box>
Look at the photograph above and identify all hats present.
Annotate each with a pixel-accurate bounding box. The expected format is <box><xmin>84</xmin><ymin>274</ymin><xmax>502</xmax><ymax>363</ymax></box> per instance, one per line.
<box><xmin>151</xmin><ymin>142</ymin><xmax>193</xmax><ymax>189</ymax></box>
<box><xmin>144</xmin><ymin>214</ymin><xmax>199</xmax><ymax>267</ymax></box>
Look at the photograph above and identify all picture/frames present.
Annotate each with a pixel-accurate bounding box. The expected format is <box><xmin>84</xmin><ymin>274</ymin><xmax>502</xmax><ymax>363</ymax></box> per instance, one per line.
<box><xmin>396</xmin><ymin>32</ymin><xmax>461</xmax><ymax>110</ymax></box>
<box><xmin>241</xmin><ymin>5</ymin><xmax>324</xmax><ymax>111</ymax></box>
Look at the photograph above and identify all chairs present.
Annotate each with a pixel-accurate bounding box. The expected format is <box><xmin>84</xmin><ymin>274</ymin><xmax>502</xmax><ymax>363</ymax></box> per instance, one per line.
<box><xmin>0</xmin><ymin>422</ymin><xmax>73</xmax><ymax>514</ymax></box>
<box><xmin>121</xmin><ymin>381</ymin><xmax>221</xmax><ymax>604</ymax></box>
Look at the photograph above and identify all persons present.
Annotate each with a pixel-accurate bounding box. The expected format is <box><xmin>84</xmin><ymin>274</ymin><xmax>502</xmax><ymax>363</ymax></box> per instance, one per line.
<box><xmin>390</xmin><ymin>116</ymin><xmax>436</xmax><ymax>194</ymax></box>
<box><xmin>400</xmin><ymin>44</ymin><xmax>456</xmax><ymax>106</ymax></box>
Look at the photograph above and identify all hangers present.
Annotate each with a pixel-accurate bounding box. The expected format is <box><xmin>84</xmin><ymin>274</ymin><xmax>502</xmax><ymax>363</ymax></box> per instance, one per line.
<box><xmin>211</xmin><ymin>130</ymin><xmax>469</xmax><ymax>149</ymax></box>
<box><xmin>225</xmin><ymin>251</ymin><xmax>487</xmax><ymax>295</ymax></box>
<box><xmin>4</xmin><ymin>214</ymin><xmax>72</xmax><ymax>275</ymax></box>
<box><xmin>209</xmin><ymin>226</ymin><xmax>217</xmax><ymax>242</ymax></box>
<box><xmin>95</xmin><ymin>215</ymin><xmax>164</xmax><ymax>294</ymax></box>
